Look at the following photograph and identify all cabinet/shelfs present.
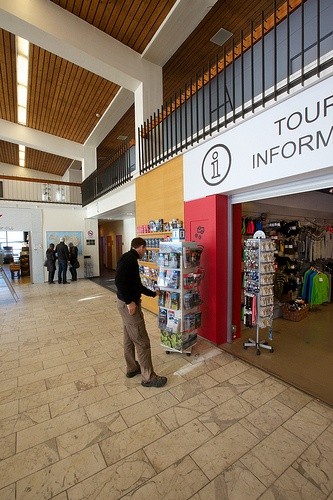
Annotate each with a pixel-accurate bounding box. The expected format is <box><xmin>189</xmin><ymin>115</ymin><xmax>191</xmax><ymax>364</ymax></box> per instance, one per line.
<box><xmin>157</xmin><ymin>240</ymin><xmax>203</xmax><ymax>357</ymax></box>
<box><xmin>136</xmin><ymin>232</ymin><xmax>173</xmax><ymax>316</ymax></box>
<box><xmin>242</xmin><ymin>238</ymin><xmax>279</xmax><ymax>355</ymax></box>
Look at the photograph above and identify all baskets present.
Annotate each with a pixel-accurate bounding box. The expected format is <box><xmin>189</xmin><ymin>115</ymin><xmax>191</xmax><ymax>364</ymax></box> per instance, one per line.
<box><xmin>282</xmin><ymin>303</ymin><xmax>309</xmax><ymax>323</ymax></box>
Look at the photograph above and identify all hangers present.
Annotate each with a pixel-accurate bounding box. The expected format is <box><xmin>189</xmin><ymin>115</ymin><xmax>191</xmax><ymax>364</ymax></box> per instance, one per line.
<box><xmin>299</xmin><ymin>216</ymin><xmax>333</xmax><ymax>276</ymax></box>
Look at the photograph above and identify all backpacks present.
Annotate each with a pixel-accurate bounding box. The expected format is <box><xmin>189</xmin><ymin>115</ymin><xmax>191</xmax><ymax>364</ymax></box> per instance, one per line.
<box><xmin>241</xmin><ymin>218</ymin><xmax>246</xmax><ymax>235</ymax></box>
<box><xmin>254</xmin><ymin>217</ymin><xmax>263</xmax><ymax>236</ymax></box>
<box><xmin>246</xmin><ymin>218</ymin><xmax>254</xmax><ymax>235</ymax></box>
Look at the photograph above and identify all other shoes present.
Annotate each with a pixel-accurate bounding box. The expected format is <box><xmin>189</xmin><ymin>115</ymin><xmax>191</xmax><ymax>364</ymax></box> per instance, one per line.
<box><xmin>141</xmin><ymin>376</ymin><xmax>167</xmax><ymax>388</ymax></box>
<box><xmin>48</xmin><ymin>281</ymin><xmax>55</xmax><ymax>284</ymax></box>
<box><xmin>126</xmin><ymin>368</ymin><xmax>142</xmax><ymax>378</ymax></box>
<box><xmin>63</xmin><ymin>282</ymin><xmax>70</xmax><ymax>284</ymax></box>
<box><xmin>70</xmin><ymin>279</ymin><xmax>77</xmax><ymax>281</ymax></box>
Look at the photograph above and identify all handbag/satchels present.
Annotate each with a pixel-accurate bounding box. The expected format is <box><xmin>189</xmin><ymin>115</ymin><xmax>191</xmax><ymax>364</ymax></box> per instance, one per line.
<box><xmin>69</xmin><ymin>260</ymin><xmax>80</xmax><ymax>269</ymax></box>
<box><xmin>43</xmin><ymin>259</ymin><xmax>53</xmax><ymax>267</ymax></box>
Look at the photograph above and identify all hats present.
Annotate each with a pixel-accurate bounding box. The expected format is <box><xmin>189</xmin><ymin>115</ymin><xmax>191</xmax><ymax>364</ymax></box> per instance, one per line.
<box><xmin>60</xmin><ymin>236</ymin><xmax>65</xmax><ymax>242</ymax></box>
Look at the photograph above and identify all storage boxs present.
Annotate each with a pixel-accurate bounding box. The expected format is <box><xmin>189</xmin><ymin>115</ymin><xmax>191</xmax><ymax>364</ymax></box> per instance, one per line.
<box><xmin>281</xmin><ymin>305</ymin><xmax>311</xmax><ymax>322</ymax></box>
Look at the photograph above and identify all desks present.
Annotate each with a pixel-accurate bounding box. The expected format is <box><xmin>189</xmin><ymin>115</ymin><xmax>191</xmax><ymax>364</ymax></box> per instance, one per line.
<box><xmin>9</xmin><ymin>264</ymin><xmax>21</xmax><ymax>279</ymax></box>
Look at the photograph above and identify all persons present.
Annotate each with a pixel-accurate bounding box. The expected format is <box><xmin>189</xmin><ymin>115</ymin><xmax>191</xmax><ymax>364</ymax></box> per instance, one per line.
<box><xmin>68</xmin><ymin>244</ymin><xmax>80</xmax><ymax>280</ymax></box>
<box><xmin>55</xmin><ymin>238</ymin><xmax>70</xmax><ymax>284</ymax></box>
<box><xmin>46</xmin><ymin>243</ymin><xmax>56</xmax><ymax>284</ymax></box>
<box><xmin>114</xmin><ymin>238</ymin><xmax>166</xmax><ymax>387</ymax></box>
